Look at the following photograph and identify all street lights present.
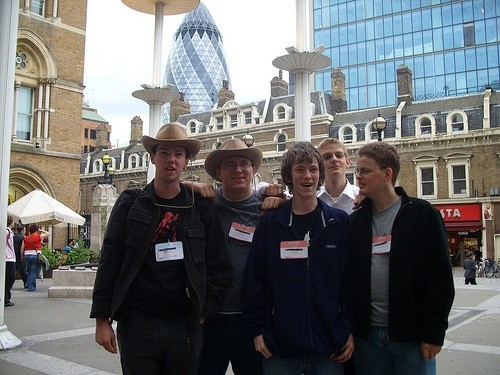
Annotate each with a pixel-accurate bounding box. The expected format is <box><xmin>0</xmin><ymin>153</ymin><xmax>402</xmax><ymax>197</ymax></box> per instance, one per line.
<box><xmin>371</xmin><ymin>111</ymin><xmax>386</xmax><ymax>141</ymax></box>
<box><xmin>102</xmin><ymin>154</ymin><xmax>114</xmax><ymax>184</ymax></box>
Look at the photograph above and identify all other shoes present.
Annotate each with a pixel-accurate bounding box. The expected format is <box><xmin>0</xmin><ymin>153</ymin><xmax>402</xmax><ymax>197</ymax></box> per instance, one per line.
<box><xmin>5</xmin><ymin>303</ymin><xmax>14</xmax><ymax>307</ymax></box>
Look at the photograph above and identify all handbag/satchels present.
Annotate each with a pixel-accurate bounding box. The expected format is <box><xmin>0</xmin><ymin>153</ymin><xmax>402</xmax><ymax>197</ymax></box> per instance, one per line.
<box><xmin>37</xmin><ymin>253</ymin><xmax>45</xmax><ymax>265</ymax></box>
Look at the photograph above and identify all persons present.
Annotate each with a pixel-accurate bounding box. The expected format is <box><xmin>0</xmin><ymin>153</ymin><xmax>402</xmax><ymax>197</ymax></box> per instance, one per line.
<box><xmin>71</xmin><ymin>238</ymin><xmax>78</xmax><ymax>249</ymax></box>
<box><xmin>88</xmin><ymin>122</ymin><xmax>233</xmax><ymax>375</ymax></box>
<box><xmin>448</xmin><ymin>244</ymin><xmax>482</xmax><ymax>266</ymax></box>
<box><xmin>20</xmin><ymin>224</ymin><xmax>51</xmax><ymax>292</ymax></box>
<box><xmin>251</xmin><ymin>137</ymin><xmax>366</xmax><ymax>215</ymax></box>
<box><xmin>463</xmin><ymin>252</ymin><xmax>478</xmax><ymax>285</ymax></box>
<box><xmin>4</xmin><ymin>215</ymin><xmax>16</xmax><ymax>307</ymax></box>
<box><xmin>13</xmin><ymin>225</ymin><xmax>29</xmax><ymax>290</ymax></box>
<box><xmin>242</xmin><ymin>140</ymin><xmax>356</xmax><ymax>375</ymax></box>
<box><xmin>63</xmin><ymin>239</ymin><xmax>74</xmax><ymax>252</ymax></box>
<box><xmin>180</xmin><ymin>138</ymin><xmax>287</xmax><ymax>375</ymax></box>
<box><xmin>349</xmin><ymin>143</ymin><xmax>456</xmax><ymax>375</ymax></box>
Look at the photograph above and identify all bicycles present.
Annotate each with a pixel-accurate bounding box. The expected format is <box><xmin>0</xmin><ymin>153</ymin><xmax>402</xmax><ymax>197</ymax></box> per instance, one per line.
<box><xmin>478</xmin><ymin>258</ymin><xmax>500</xmax><ymax>278</ymax></box>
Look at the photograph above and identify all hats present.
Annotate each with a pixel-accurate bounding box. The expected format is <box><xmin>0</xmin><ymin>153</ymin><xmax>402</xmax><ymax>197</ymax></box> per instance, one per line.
<box><xmin>204</xmin><ymin>139</ymin><xmax>263</xmax><ymax>182</ymax></box>
<box><xmin>141</xmin><ymin>122</ymin><xmax>201</xmax><ymax>158</ymax></box>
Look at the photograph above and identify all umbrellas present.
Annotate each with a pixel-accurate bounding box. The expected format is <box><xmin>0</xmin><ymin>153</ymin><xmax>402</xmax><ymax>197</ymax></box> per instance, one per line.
<box><xmin>7</xmin><ymin>187</ymin><xmax>86</xmax><ymax>282</ymax></box>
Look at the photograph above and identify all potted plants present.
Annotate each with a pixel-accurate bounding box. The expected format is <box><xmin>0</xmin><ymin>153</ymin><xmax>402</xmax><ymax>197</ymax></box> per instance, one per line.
<box><xmin>42</xmin><ymin>246</ymin><xmax>62</xmax><ymax>278</ymax></box>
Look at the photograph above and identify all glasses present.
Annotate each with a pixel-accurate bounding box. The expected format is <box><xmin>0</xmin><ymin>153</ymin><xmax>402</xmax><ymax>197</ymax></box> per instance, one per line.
<box><xmin>354</xmin><ymin>166</ymin><xmax>385</xmax><ymax>175</ymax></box>
